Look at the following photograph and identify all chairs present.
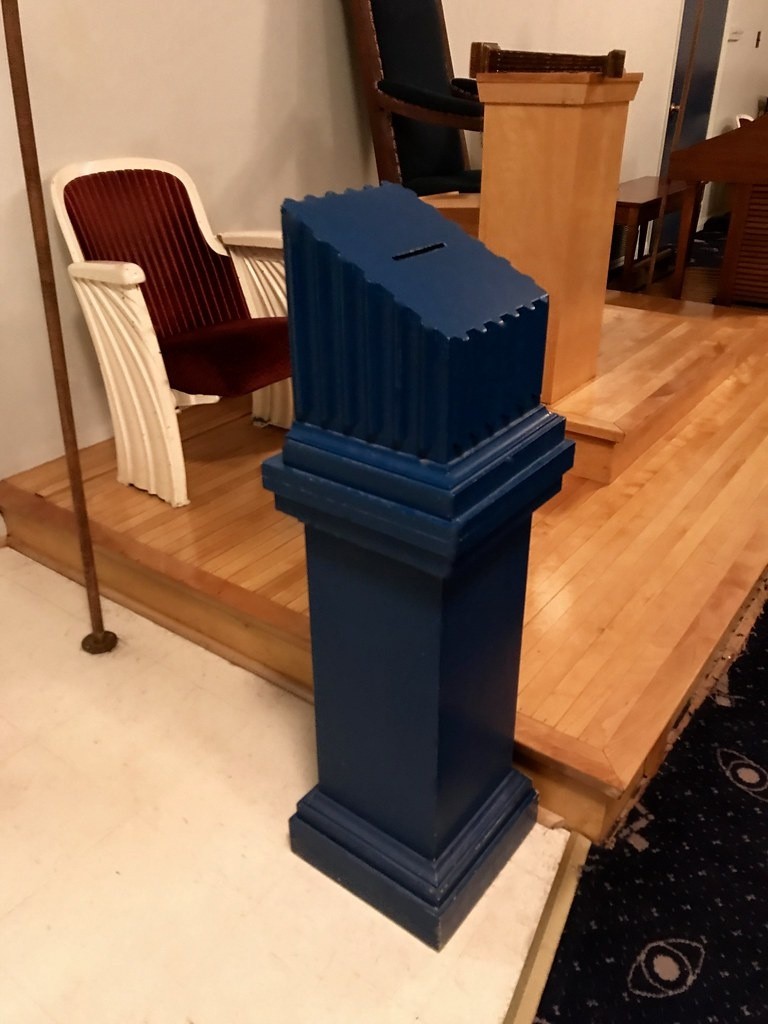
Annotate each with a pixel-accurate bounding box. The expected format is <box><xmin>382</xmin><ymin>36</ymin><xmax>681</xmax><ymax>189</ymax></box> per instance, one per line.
<box><xmin>52</xmin><ymin>154</ymin><xmax>295</xmax><ymax>505</ymax></box>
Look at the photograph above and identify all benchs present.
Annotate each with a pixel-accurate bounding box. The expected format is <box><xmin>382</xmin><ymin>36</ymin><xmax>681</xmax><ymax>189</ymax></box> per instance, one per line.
<box><xmin>605</xmin><ymin>176</ymin><xmax>709</xmax><ymax>292</ymax></box>
